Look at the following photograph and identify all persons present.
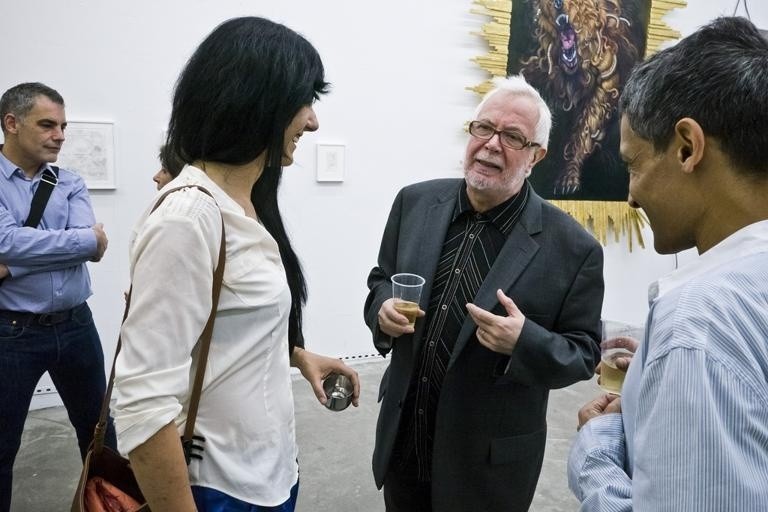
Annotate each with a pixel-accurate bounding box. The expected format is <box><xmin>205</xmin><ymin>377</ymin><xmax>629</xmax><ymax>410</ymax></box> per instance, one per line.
<box><xmin>0</xmin><ymin>83</ymin><xmax>117</xmax><ymax>512</ymax></box>
<box><xmin>364</xmin><ymin>73</ymin><xmax>605</xmax><ymax>512</ymax></box>
<box><xmin>152</xmin><ymin>143</ymin><xmax>182</xmax><ymax>192</ymax></box>
<box><xmin>568</xmin><ymin>17</ymin><xmax>768</xmax><ymax>512</ymax></box>
<box><xmin>111</xmin><ymin>17</ymin><xmax>360</xmax><ymax>512</ymax></box>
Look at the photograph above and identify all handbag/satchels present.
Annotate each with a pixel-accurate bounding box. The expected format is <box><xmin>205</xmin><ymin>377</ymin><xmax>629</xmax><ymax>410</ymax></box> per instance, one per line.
<box><xmin>71</xmin><ymin>445</ymin><xmax>152</xmax><ymax>512</ymax></box>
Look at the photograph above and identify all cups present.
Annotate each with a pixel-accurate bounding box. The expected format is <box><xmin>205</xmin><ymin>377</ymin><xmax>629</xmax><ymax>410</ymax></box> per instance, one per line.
<box><xmin>391</xmin><ymin>273</ymin><xmax>426</xmax><ymax>330</ymax></box>
<box><xmin>599</xmin><ymin>318</ymin><xmax>644</xmax><ymax>395</ymax></box>
<box><xmin>322</xmin><ymin>374</ymin><xmax>354</xmax><ymax>411</ymax></box>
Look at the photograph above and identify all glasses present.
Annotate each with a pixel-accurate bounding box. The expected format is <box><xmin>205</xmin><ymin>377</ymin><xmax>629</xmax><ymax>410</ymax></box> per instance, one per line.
<box><xmin>469</xmin><ymin>120</ymin><xmax>541</xmax><ymax>150</ymax></box>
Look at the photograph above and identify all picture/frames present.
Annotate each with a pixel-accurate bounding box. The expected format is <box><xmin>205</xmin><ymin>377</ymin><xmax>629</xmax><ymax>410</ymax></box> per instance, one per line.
<box><xmin>48</xmin><ymin>120</ymin><xmax>117</xmax><ymax>191</ymax></box>
<box><xmin>316</xmin><ymin>143</ymin><xmax>346</xmax><ymax>183</ymax></box>
